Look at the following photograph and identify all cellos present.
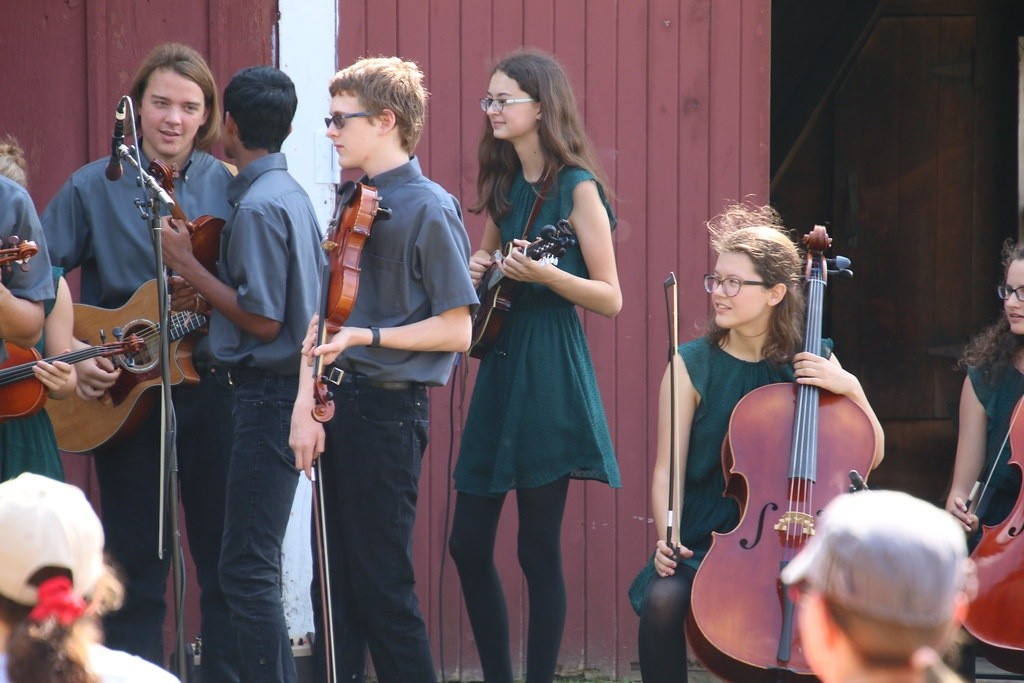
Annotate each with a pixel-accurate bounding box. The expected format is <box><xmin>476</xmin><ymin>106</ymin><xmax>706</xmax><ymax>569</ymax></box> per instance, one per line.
<box><xmin>686</xmin><ymin>223</ymin><xmax>877</xmax><ymax>683</ymax></box>
<box><xmin>956</xmin><ymin>392</ymin><xmax>1024</xmax><ymax>674</ymax></box>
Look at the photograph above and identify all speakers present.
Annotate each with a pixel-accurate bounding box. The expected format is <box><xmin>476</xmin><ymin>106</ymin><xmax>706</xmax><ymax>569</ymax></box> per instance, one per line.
<box><xmin>171</xmin><ymin>643</ymin><xmax>318</xmax><ymax>683</ymax></box>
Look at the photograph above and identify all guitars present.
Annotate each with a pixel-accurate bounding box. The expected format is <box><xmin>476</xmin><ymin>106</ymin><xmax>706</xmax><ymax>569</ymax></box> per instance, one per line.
<box><xmin>43</xmin><ymin>272</ymin><xmax>208</xmax><ymax>456</ymax></box>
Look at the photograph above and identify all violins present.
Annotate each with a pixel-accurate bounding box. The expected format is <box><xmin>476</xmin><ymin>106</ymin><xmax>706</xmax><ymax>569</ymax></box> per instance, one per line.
<box><xmin>467</xmin><ymin>217</ymin><xmax>576</xmax><ymax>361</ymax></box>
<box><xmin>0</xmin><ymin>327</ymin><xmax>148</xmax><ymax>425</ymax></box>
<box><xmin>309</xmin><ymin>182</ymin><xmax>393</xmax><ymax>425</ymax></box>
<box><xmin>0</xmin><ymin>234</ymin><xmax>38</xmax><ymax>280</ymax></box>
<box><xmin>149</xmin><ymin>156</ymin><xmax>226</xmax><ymax>280</ymax></box>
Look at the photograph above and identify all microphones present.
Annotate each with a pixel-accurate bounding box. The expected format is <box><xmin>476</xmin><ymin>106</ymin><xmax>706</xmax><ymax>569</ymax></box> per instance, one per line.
<box><xmin>105</xmin><ymin>100</ymin><xmax>126</xmax><ymax>181</ymax></box>
<box><xmin>539</xmin><ymin>225</ymin><xmax>562</xmax><ymax>243</ymax></box>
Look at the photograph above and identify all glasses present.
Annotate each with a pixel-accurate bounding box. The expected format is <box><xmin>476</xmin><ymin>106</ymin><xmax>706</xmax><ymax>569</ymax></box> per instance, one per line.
<box><xmin>325</xmin><ymin>111</ymin><xmax>379</xmax><ymax>130</ymax></box>
<box><xmin>998</xmin><ymin>285</ymin><xmax>1024</xmax><ymax>302</ymax></box>
<box><xmin>478</xmin><ymin>97</ymin><xmax>537</xmax><ymax>113</ymax></box>
<box><xmin>703</xmin><ymin>271</ymin><xmax>770</xmax><ymax>297</ymax></box>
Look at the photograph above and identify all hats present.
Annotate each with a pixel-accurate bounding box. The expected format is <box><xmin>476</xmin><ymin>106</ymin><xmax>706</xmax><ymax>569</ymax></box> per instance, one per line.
<box><xmin>781</xmin><ymin>490</ymin><xmax>968</xmax><ymax>626</ymax></box>
<box><xmin>0</xmin><ymin>472</ymin><xmax>103</xmax><ymax>607</ymax></box>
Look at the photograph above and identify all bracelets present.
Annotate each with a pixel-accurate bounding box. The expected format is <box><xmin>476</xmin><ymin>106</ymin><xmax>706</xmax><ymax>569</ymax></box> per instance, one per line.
<box><xmin>366</xmin><ymin>326</ymin><xmax>380</xmax><ymax>349</ymax></box>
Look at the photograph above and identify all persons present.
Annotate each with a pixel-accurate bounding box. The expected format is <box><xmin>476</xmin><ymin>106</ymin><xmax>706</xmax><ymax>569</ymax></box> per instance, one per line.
<box><xmin>628</xmin><ymin>205</ymin><xmax>886</xmax><ymax>683</ymax></box>
<box><xmin>161</xmin><ymin>65</ymin><xmax>324</xmax><ymax>683</ymax></box>
<box><xmin>945</xmin><ymin>241</ymin><xmax>1024</xmax><ymax>556</ymax></box>
<box><xmin>289</xmin><ymin>56</ymin><xmax>481</xmax><ymax>683</ymax></box>
<box><xmin>39</xmin><ymin>43</ymin><xmax>233</xmax><ymax>683</ymax></box>
<box><xmin>449</xmin><ymin>53</ymin><xmax>623</xmax><ymax>683</ymax></box>
<box><xmin>780</xmin><ymin>490</ymin><xmax>976</xmax><ymax>683</ymax></box>
<box><xmin>0</xmin><ymin>137</ymin><xmax>182</xmax><ymax>683</ymax></box>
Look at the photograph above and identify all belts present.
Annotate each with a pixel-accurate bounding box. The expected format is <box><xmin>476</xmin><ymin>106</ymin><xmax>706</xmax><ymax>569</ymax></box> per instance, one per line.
<box><xmin>324</xmin><ymin>365</ymin><xmax>414</xmax><ymax>390</ymax></box>
